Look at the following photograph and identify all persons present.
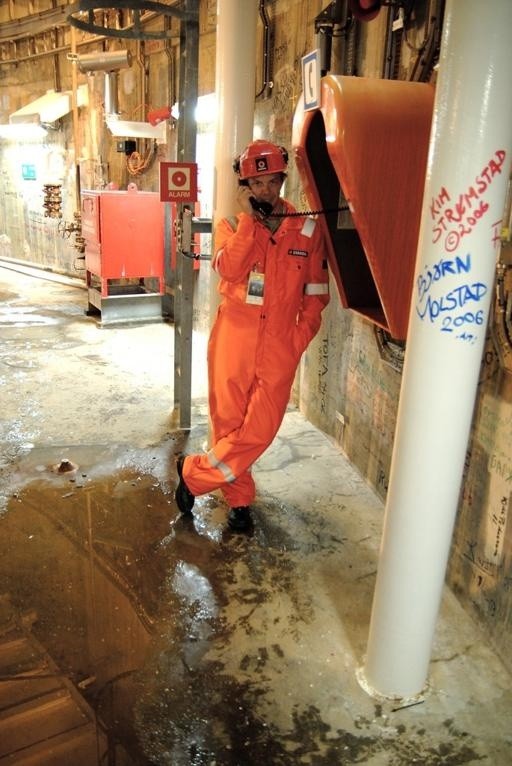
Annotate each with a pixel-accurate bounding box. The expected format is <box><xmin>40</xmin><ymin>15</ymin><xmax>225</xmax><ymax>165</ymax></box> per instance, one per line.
<box><xmin>173</xmin><ymin>140</ymin><xmax>332</xmax><ymax>536</ymax></box>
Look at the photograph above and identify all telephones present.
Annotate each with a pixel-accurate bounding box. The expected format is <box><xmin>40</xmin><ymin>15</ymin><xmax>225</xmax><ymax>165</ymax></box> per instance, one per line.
<box><xmin>239</xmin><ymin>178</ymin><xmax>273</xmax><ymax>216</ymax></box>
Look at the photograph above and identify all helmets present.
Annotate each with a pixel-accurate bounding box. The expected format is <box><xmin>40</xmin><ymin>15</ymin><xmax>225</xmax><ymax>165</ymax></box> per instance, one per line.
<box><xmin>238</xmin><ymin>138</ymin><xmax>287</xmax><ymax>181</ymax></box>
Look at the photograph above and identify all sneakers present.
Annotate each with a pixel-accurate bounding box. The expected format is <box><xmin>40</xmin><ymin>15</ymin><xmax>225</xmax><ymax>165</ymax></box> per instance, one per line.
<box><xmin>175</xmin><ymin>454</ymin><xmax>196</xmax><ymax>513</ymax></box>
<box><xmin>227</xmin><ymin>506</ymin><xmax>253</xmax><ymax>531</ymax></box>
